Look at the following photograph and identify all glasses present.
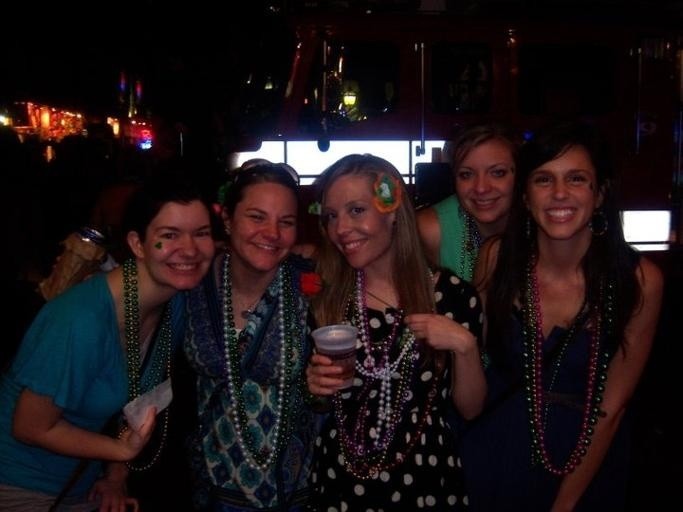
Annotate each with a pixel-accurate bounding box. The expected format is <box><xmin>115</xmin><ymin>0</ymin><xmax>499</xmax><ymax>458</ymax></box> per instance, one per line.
<box><xmin>241</xmin><ymin>158</ymin><xmax>301</xmax><ymax>187</ymax></box>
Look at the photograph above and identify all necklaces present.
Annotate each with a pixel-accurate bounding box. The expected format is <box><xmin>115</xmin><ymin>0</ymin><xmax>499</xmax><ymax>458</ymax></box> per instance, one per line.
<box><xmin>515</xmin><ymin>238</ymin><xmax>620</xmax><ymax>479</ymax></box>
<box><xmin>218</xmin><ymin>244</ymin><xmax>304</xmax><ymax>469</ymax></box>
<box><xmin>321</xmin><ymin>263</ymin><xmax>453</xmax><ymax>481</ymax></box>
<box><xmin>457</xmin><ymin>207</ymin><xmax>485</xmax><ymax>284</ymax></box>
<box><xmin>116</xmin><ymin>257</ymin><xmax>175</xmax><ymax>471</ymax></box>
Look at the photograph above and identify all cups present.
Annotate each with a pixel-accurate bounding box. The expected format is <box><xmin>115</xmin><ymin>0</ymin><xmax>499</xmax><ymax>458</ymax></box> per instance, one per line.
<box><xmin>311</xmin><ymin>324</ymin><xmax>358</xmax><ymax>391</ymax></box>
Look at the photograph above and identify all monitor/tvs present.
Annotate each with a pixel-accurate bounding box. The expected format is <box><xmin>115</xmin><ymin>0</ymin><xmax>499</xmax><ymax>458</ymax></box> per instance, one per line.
<box><xmin>617</xmin><ymin>207</ymin><xmax>674</xmax><ymax>244</ymax></box>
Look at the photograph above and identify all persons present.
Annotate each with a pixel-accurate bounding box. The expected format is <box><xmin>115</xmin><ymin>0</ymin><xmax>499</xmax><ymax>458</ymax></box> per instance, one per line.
<box><xmin>0</xmin><ymin>178</ymin><xmax>214</xmax><ymax>511</ymax></box>
<box><xmin>467</xmin><ymin>113</ymin><xmax>663</xmax><ymax>508</ymax></box>
<box><xmin>1</xmin><ymin>122</ymin><xmax>144</xmax><ymax>364</ymax></box>
<box><xmin>53</xmin><ymin>157</ymin><xmax>334</xmax><ymax>511</ymax></box>
<box><xmin>407</xmin><ymin>127</ymin><xmax>538</xmax><ymax>306</ymax></box>
<box><xmin>305</xmin><ymin>151</ymin><xmax>490</xmax><ymax>512</ymax></box>
